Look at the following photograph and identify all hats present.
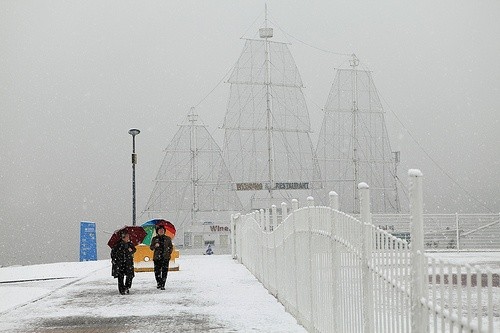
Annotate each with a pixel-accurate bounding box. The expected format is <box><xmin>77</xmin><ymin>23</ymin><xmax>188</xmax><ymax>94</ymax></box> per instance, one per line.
<box><xmin>156</xmin><ymin>226</ymin><xmax>166</xmax><ymax>234</ymax></box>
<box><xmin>120</xmin><ymin>230</ymin><xmax>128</xmax><ymax>238</ymax></box>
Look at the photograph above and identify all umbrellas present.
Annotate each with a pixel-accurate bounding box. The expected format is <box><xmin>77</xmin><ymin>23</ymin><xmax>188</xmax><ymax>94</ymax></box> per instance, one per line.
<box><xmin>140</xmin><ymin>219</ymin><xmax>176</xmax><ymax>245</ymax></box>
<box><xmin>107</xmin><ymin>226</ymin><xmax>147</xmax><ymax>249</ymax></box>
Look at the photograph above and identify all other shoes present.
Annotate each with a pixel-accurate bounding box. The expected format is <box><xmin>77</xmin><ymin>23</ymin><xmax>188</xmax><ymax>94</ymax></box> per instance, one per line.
<box><xmin>157</xmin><ymin>286</ymin><xmax>161</xmax><ymax>288</ymax></box>
<box><xmin>161</xmin><ymin>287</ymin><xmax>165</xmax><ymax>290</ymax></box>
<box><xmin>120</xmin><ymin>286</ymin><xmax>129</xmax><ymax>295</ymax></box>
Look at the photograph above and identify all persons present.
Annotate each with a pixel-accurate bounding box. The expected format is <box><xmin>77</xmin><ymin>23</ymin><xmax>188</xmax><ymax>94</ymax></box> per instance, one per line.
<box><xmin>150</xmin><ymin>226</ymin><xmax>172</xmax><ymax>290</ymax></box>
<box><xmin>111</xmin><ymin>232</ymin><xmax>136</xmax><ymax>295</ymax></box>
<box><xmin>206</xmin><ymin>244</ymin><xmax>213</xmax><ymax>254</ymax></box>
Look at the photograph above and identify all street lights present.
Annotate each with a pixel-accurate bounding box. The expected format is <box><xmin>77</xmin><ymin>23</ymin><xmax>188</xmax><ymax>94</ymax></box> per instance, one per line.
<box><xmin>128</xmin><ymin>129</ymin><xmax>141</xmax><ymax>226</ymax></box>
